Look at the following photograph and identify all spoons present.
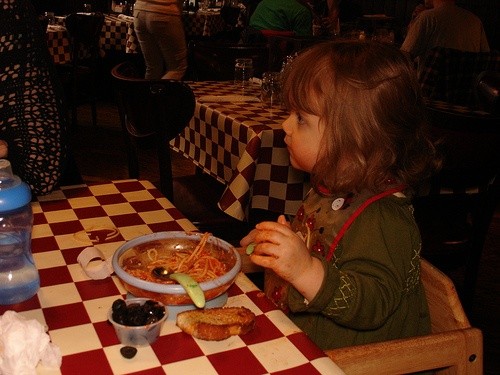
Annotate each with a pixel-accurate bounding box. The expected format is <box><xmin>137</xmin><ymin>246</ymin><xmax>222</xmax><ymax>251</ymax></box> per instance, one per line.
<box><xmin>152</xmin><ymin>267</ymin><xmax>206</xmax><ymax>309</ymax></box>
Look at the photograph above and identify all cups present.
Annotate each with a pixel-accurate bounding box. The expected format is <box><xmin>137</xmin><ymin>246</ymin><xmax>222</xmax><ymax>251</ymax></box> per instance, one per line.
<box><xmin>233</xmin><ymin>58</ymin><xmax>253</xmax><ymax>89</ymax></box>
<box><xmin>313</xmin><ymin>25</ymin><xmax>321</xmax><ymax>36</ymax></box>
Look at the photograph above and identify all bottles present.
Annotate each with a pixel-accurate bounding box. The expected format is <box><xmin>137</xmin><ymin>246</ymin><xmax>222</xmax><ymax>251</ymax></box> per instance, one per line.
<box><xmin>261</xmin><ymin>73</ymin><xmax>285</xmax><ymax>109</ymax></box>
<box><xmin>0</xmin><ymin>159</ymin><xmax>42</xmax><ymax>306</ymax></box>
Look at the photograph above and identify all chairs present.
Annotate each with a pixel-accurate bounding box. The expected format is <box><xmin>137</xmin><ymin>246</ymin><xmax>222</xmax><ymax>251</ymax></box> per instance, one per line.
<box><xmin>233</xmin><ymin>243</ymin><xmax>483</xmax><ymax>375</ymax></box>
<box><xmin>188</xmin><ymin>40</ymin><xmax>268</xmax><ymax>79</ymax></box>
<box><xmin>413</xmin><ymin>50</ymin><xmax>500</xmax><ymax>301</ymax></box>
<box><xmin>270</xmin><ymin>35</ymin><xmax>318</xmax><ymax>72</ymax></box>
<box><xmin>52</xmin><ymin>12</ymin><xmax>106</xmax><ymax>127</ymax></box>
<box><xmin>112</xmin><ymin>60</ymin><xmax>243</xmax><ymax>224</ymax></box>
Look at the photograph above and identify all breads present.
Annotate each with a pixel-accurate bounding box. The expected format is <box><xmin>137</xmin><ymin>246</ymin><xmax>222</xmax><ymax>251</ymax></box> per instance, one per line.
<box><xmin>177</xmin><ymin>306</ymin><xmax>254</xmax><ymax>342</ymax></box>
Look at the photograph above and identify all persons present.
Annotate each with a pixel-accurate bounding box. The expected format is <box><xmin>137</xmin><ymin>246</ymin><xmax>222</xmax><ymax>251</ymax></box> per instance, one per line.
<box><xmin>132</xmin><ymin>1</ymin><xmax>490</xmax><ymax>95</ymax></box>
<box><xmin>0</xmin><ymin>0</ymin><xmax>61</xmax><ymax>195</ymax></box>
<box><xmin>240</xmin><ymin>39</ymin><xmax>434</xmax><ymax>375</ymax></box>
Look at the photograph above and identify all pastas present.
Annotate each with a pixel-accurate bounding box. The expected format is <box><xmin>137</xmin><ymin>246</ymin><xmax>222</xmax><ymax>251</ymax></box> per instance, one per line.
<box><xmin>123</xmin><ymin>231</ymin><xmax>228</xmax><ymax>306</ymax></box>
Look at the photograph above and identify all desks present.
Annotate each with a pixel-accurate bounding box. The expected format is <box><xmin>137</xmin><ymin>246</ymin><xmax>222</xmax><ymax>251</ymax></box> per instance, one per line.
<box><xmin>174</xmin><ymin>80</ymin><xmax>486</xmax><ymax>220</ymax></box>
<box><xmin>0</xmin><ymin>176</ymin><xmax>345</xmax><ymax>375</ymax></box>
<box><xmin>47</xmin><ymin>6</ymin><xmax>234</xmax><ymax>63</ymax></box>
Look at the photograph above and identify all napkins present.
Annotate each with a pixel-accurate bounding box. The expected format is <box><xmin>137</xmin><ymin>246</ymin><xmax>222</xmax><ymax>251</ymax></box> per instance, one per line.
<box><xmin>0</xmin><ymin>311</ymin><xmax>61</xmax><ymax>375</ymax></box>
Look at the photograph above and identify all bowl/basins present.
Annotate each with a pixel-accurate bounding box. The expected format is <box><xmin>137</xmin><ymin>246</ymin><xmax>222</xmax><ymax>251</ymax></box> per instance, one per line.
<box><xmin>111</xmin><ymin>231</ymin><xmax>242</xmax><ymax>318</ymax></box>
<box><xmin>107</xmin><ymin>298</ymin><xmax>168</xmax><ymax>346</ymax></box>
